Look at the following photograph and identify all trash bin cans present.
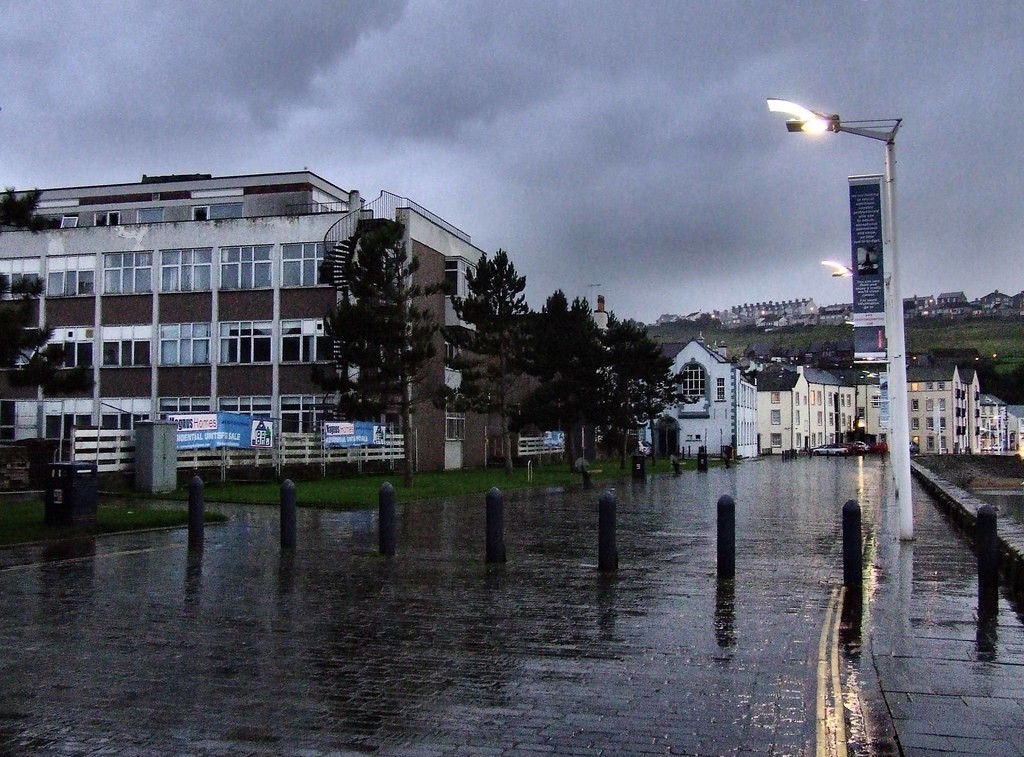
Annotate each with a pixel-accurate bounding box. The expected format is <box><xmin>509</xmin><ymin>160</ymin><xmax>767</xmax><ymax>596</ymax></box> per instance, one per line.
<box><xmin>46</xmin><ymin>461</ymin><xmax>99</xmax><ymax>525</ymax></box>
<box><xmin>697</xmin><ymin>453</ymin><xmax>709</xmax><ymax>471</ymax></box>
<box><xmin>631</xmin><ymin>453</ymin><xmax>644</xmax><ymax>477</ymax></box>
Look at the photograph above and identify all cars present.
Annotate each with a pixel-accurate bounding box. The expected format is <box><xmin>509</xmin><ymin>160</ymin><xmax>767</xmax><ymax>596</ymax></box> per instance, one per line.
<box><xmin>812</xmin><ymin>441</ymin><xmax>919</xmax><ymax>456</ymax></box>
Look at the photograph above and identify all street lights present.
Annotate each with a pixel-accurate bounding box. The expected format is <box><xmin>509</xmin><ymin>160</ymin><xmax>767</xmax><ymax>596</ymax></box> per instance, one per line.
<box><xmin>766</xmin><ymin>98</ymin><xmax>913</xmax><ymax>541</ymax></box>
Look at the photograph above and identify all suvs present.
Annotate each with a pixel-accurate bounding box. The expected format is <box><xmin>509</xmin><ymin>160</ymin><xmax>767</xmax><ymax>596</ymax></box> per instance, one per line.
<box><xmin>638</xmin><ymin>441</ymin><xmax>653</xmax><ymax>456</ymax></box>
